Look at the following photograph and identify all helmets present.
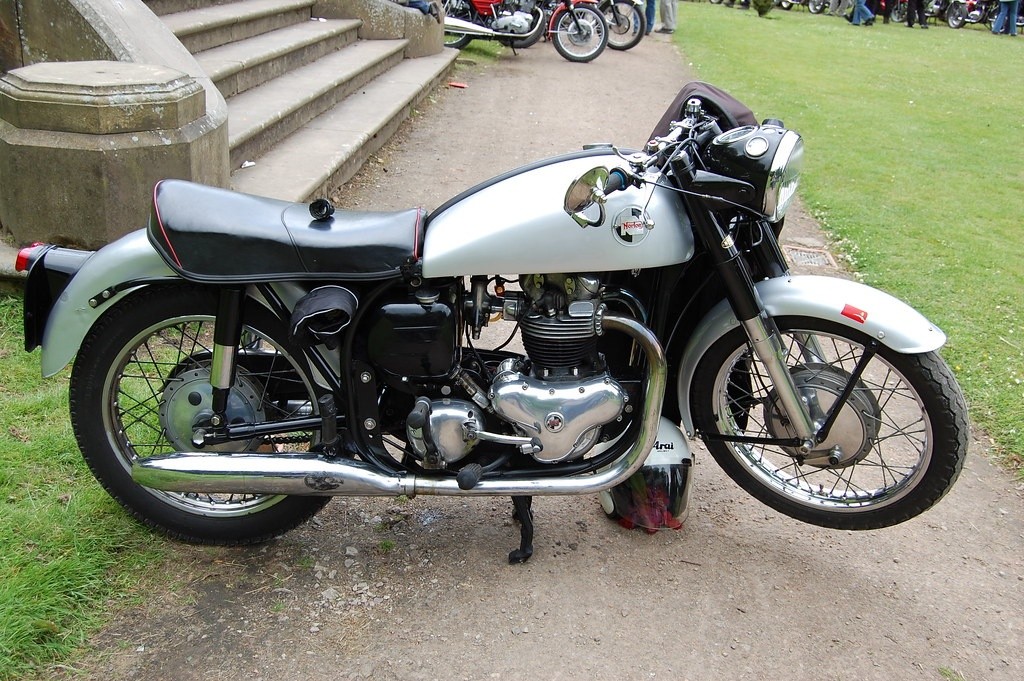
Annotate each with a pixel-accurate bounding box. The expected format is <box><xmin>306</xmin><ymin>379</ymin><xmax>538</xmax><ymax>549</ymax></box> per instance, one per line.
<box><xmin>599</xmin><ymin>416</ymin><xmax>695</xmax><ymax>534</ymax></box>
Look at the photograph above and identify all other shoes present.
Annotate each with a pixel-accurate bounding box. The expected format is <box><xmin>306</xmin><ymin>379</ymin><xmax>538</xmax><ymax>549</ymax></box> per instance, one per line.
<box><xmin>849</xmin><ymin>23</ymin><xmax>859</xmax><ymax>26</ymax></box>
<box><xmin>992</xmin><ymin>31</ymin><xmax>999</xmax><ymax>34</ymax></box>
<box><xmin>921</xmin><ymin>25</ymin><xmax>928</xmax><ymax>29</ymax></box>
<box><xmin>654</xmin><ymin>28</ymin><xmax>674</xmax><ymax>34</ymax></box>
<box><xmin>646</xmin><ymin>32</ymin><xmax>650</xmax><ymax>36</ymax></box>
<box><xmin>905</xmin><ymin>24</ymin><xmax>913</xmax><ymax>28</ymax></box>
<box><xmin>864</xmin><ymin>17</ymin><xmax>874</xmax><ymax>25</ymax></box>
<box><xmin>632</xmin><ymin>31</ymin><xmax>636</xmax><ymax>36</ymax></box>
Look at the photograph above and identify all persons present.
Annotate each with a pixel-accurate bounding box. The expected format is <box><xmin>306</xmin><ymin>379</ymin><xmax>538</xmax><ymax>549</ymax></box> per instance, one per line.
<box><xmin>656</xmin><ymin>0</ymin><xmax>1018</xmax><ymax>36</ymax></box>
<box><xmin>632</xmin><ymin>0</ymin><xmax>654</xmax><ymax>35</ymax></box>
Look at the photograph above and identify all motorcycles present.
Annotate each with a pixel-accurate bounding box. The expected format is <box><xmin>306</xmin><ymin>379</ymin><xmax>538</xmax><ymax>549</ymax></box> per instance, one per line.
<box><xmin>441</xmin><ymin>0</ymin><xmax>645</xmax><ymax>63</ymax></box>
<box><xmin>780</xmin><ymin>0</ymin><xmax>1024</xmax><ymax>34</ymax></box>
<box><xmin>13</xmin><ymin>97</ymin><xmax>968</xmax><ymax>566</ymax></box>
